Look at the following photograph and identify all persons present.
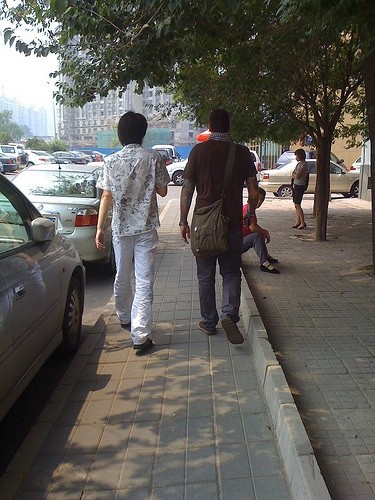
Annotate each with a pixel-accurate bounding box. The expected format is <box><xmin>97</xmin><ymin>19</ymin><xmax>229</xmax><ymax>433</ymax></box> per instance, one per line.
<box><xmin>241</xmin><ymin>187</ymin><xmax>280</xmax><ymax>274</ymax></box>
<box><xmin>292</xmin><ymin>148</ymin><xmax>309</xmax><ymax>230</ymax></box>
<box><xmin>179</xmin><ymin>109</ymin><xmax>259</xmax><ymax>344</ymax></box>
<box><xmin>95</xmin><ymin>111</ymin><xmax>171</xmax><ymax>349</ymax></box>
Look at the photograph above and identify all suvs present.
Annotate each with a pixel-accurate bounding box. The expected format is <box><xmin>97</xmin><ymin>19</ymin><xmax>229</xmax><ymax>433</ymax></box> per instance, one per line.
<box><xmin>273</xmin><ymin>149</ymin><xmax>347</xmax><ymax>173</ymax></box>
<box><xmin>166</xmin><ymin>150</ymin><xmax>262</xmax><ymax>188</ymax></box>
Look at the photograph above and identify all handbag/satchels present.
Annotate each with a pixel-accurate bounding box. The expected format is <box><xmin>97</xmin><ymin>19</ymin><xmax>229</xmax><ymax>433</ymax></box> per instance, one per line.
<box><xmin>191</xmin><ymin>199</ymin><xmax>230</xmax><ymax>258</ymax></box>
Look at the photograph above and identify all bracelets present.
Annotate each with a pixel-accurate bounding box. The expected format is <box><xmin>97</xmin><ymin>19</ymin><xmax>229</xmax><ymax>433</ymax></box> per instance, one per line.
<box><xmin>179</xmin><ymin>222</ymin><xmax>188</xmax><ymax>226</ymax></box>
<box><xmin>247</xmin><ymin>211</ymin><xmax>255</xmax><ymax>217</ymax></box>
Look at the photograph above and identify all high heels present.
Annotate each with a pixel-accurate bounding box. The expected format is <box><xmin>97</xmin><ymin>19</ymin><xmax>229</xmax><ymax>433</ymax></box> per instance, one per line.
<box><xmin>298</xmin><ymin>223</ymin><xmax>307</xmax><ymax>229</ymax></box>
<box><xmin>292</xmin><ymin>222</ymin><xmax>301</xmax><ymax>228</ymax></box>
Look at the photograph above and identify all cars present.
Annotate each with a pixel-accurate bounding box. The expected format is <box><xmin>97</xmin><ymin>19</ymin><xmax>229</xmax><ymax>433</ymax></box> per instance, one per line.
<box><xmin>0</xmin><ymin>172</ymin><xmax>86</xmax><ymax>422</ymax></box>
<box><xmin>0</xmin><ymin>163</ymin><xmax>116</xmax><ymax>278</ymax></box>
<box><xmin>0</xmin><ymin>142</ymin><xmax>182</xmax><ymax>193</ymax></box>
<box><xmin>348</xmin><ymin>154</ymin><xmax>361</xmax><ymax>174</ymax></box>
<box><xmin>258</xmin><ymin>151</ymin><xmax>362</xmax><ymax>198</ymax></box>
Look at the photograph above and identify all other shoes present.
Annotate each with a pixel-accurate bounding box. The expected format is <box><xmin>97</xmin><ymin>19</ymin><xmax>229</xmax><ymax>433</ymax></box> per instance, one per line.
<box><xmin>121</xmin><ymin>321</ymin><xmax>131</xmax><ymax>327</ymax></box>
<box><xmin>260</xmin><ymin>263</ymin><xmax>279</xmax><ymax>273</ymax></box>
<box><xmin>268</xmin><ymin>256</ymin><xmax>278</xmax><ymax>263</ymax></box>
<box><xmin>198</xmin><ymin>321</ymin><xmax>217</xmax><ymax>334</ymax></box>
<box><xmin>134</xmin><ymin>338</ymin><xmax>152</xmax><ymax>349</ymax></box>
<box><xmin>220</xmin><ymin>315</ymin><xmax>245</xmax><ymax>344</ymax></box>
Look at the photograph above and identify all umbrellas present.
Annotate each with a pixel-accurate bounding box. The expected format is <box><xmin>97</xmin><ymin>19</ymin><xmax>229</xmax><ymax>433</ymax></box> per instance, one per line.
<box><xmin>196</xmin><ymin>129</ymin><xmax>212</xmax><ymax>142</ymax></box>
